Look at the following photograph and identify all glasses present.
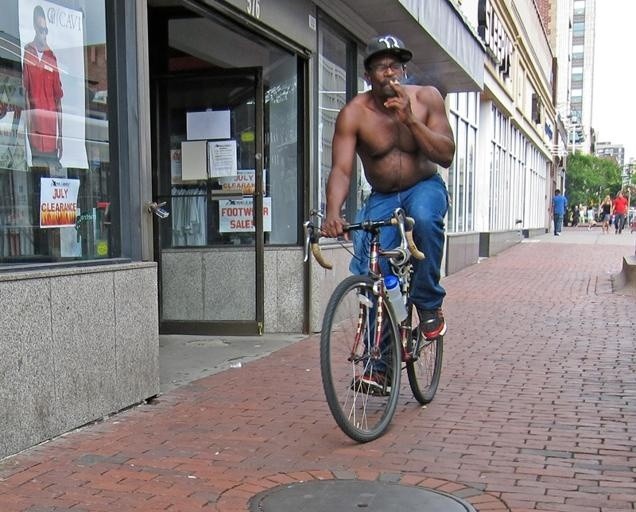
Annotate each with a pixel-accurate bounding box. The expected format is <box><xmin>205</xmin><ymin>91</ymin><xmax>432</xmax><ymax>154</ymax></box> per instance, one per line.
<box><xmin>368</xmin><ymin>61</ymin><xmax>407</xmax><ymax>70</ymax></box>
<box><xmin>34</xmin><ymin>25</ymin><xmax>49</xmax><ymax>34</ymax></box>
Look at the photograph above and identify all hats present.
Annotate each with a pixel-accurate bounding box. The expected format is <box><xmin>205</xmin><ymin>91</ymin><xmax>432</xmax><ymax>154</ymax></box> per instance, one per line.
<box><xmin>362</xmin><ymin>33</ymin><xmax>413</xmax><ymax>70</ymax></box>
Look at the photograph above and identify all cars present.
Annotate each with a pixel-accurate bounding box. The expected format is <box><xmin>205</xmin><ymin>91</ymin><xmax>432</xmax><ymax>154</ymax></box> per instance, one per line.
<box><xmin>625</xmin><ymin>206</ymin><xmax>636</xmax><ymax>234</ymax></box>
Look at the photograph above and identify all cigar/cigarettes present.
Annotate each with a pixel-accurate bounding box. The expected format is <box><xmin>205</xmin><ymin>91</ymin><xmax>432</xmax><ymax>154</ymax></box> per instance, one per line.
<box><xmin>392</xmin><ymin>81</ymin><xmax>401</xmax><ymax>86</ymax></box>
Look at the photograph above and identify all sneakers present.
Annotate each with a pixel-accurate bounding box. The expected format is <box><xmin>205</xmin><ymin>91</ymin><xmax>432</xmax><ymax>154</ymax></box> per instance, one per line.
<box><xmin>350</xmin><ymin>371</ymin><xmax>393</xmax><ymax>398</ymax></box>
<box><xmin>415</xmin><ymin>304</ymin><xmax>447</xmax><ymax>341</ymax></box>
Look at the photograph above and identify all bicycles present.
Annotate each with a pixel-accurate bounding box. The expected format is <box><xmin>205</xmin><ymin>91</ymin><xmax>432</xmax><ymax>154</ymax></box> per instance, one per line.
<box><xmin>301</xmin><ymin>204</ymin><xmax>453</xmax><ymax>441</ymax></box>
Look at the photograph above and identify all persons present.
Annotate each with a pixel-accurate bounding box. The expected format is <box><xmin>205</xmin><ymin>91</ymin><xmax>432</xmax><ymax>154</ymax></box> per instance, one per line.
<box><xmin>319</xmin><ymin>35</ymin><xmax>458</xmax><ymax>393</ymax></box>
<box><xmin>19</xmin><ymin>4</ymin><xmax>63</xmax><ymax>169</ymax></box>
<box><xmin>569</xmin><ymin>195</ymin><xmax>614</xmax><ymax>230</ymax></box>
<box><xmin>612</xmin><ymin>191</ymin><xmax>628</xmax><ymax>233</ymax></box>
<box><xmin>548</xmin><ymin>190</ymin><xmax>568</xmax><ymax>235</ymax></box>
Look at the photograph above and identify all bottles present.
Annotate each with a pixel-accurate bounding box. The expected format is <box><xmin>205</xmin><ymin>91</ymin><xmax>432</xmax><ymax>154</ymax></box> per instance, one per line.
<box><xmin>383</xmin><ymin>276</ymin><xmax>408</xmax><ymax>324</ymax></box>
